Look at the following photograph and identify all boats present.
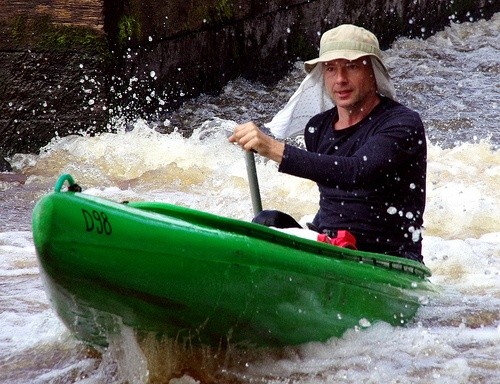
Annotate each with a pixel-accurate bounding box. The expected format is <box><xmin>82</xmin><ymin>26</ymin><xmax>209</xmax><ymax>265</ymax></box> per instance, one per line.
<box><xmin>29</xmin><ymin>173</ymin><xmax>434</xmax><ymax>351</ymax></box>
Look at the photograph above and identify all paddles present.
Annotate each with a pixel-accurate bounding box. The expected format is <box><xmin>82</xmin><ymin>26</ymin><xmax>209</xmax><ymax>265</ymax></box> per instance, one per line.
<box><xmin>240</xmin><ymin>134</ymin><xmax>264</xmax><ymax>219</ymax></box>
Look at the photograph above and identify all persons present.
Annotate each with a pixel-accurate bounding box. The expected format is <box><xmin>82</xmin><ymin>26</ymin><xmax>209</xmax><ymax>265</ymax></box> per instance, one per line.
<box><xmin>229</xmin><ymin>24</ymin><xmax>427</xmax><ymax>267</ymax></box>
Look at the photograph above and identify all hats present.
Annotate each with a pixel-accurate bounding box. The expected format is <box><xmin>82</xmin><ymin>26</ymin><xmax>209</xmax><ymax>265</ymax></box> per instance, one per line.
<box><xmin>304</xmin><ymin>24</ymin><xmax>388</xmax><ymax>76</ymax></box>
